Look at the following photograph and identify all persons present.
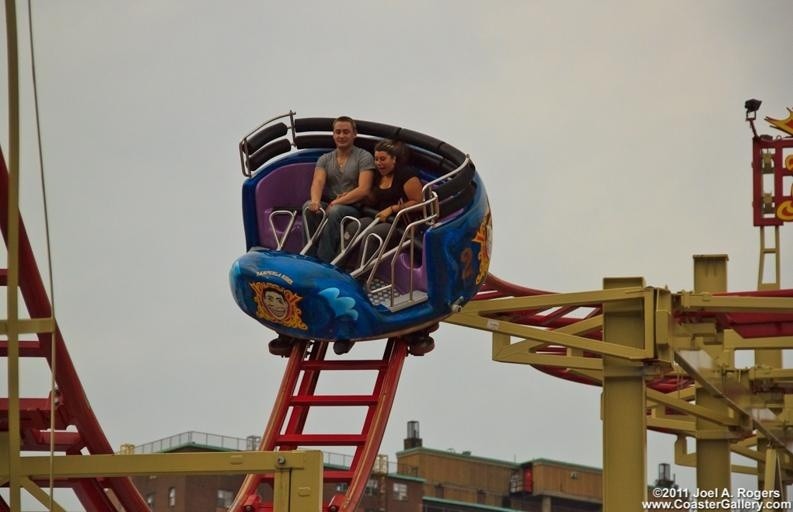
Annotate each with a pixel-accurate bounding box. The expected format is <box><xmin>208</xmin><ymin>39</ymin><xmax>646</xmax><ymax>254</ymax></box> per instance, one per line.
<box><xmin>332</xmin><ymin>137</ymin><xmax>425</xmax><ymax>283</ymax></box>
<box><xmin>296</xmin><ymin>113</ymin><xmax>374</xmax><ymax>264</ymax></box>
